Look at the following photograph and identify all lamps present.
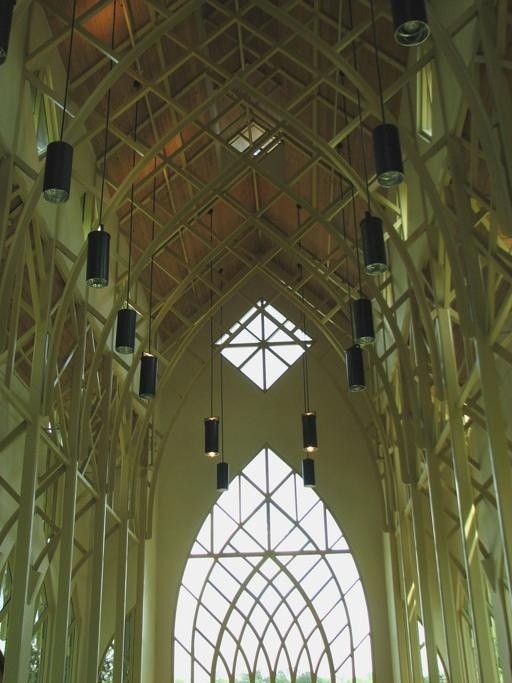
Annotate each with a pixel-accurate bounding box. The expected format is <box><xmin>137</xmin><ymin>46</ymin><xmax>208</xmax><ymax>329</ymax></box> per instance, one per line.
<box><xmin>139</xmin><ymin>43</ymin><xmax>157</xmax><ymax>398</ymax></box>
<box><xmin>343</xmin><ymin>0</ymin><xmax>430</xmax><ymax>393</ymax></box>
<box><xmin>43</xmin><ymin>1</ymin><xmax>74</xmax><ymax>203</ymax></box>
<box><xmin>217</xmin><ymin>268</ymin><xmax>228</xmax><ymax>492</ymax></box>
<box><xmin>298</xmin><ymin>264</ymin><xmax>314</xmax><ymax>488</ymax></box>
<box><xmin>204</xmin><ymin>209</ymin><xmax>219</xmax><ymax>458</ymax></box>
<box><xmin>297</xmin><ymin>204</ymin><xmax>317</xmax><ymax>453</ymax></box>
<box><xmin>116</xmin><ymin>1</ymin><xmax>139</xmax><ymax>355</ymax></box>
<box><xmin>85</xmin><ymin>1</ymin><xmax>115</xmax><ymax>288</ymax></box>
